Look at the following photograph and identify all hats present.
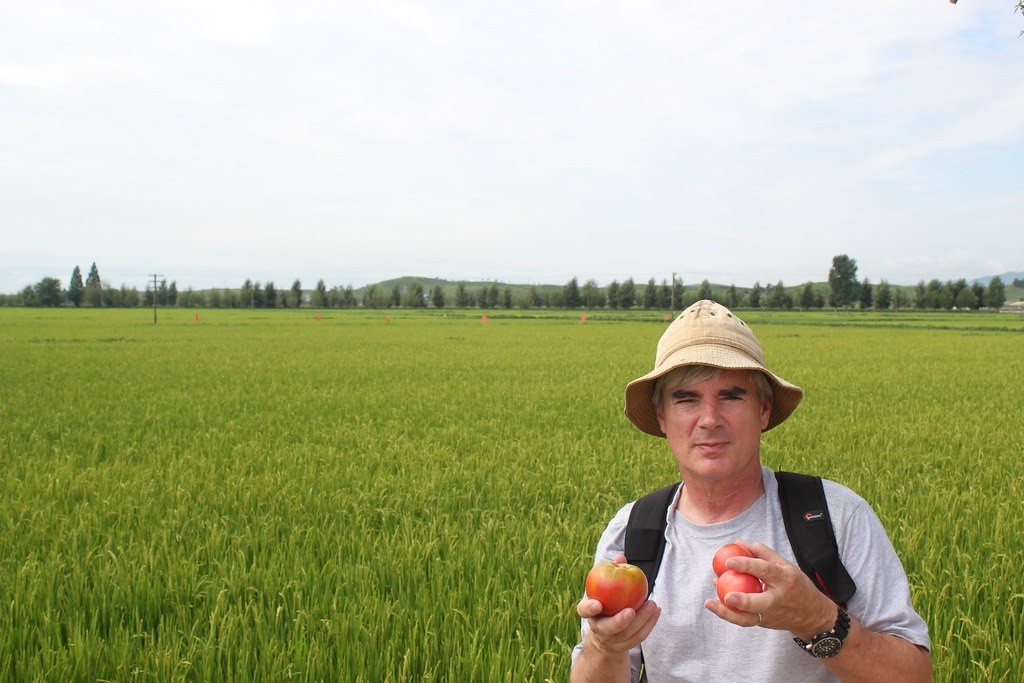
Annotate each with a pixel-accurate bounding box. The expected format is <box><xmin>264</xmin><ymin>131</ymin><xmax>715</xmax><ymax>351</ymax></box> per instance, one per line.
<box><xmin>623</xmin><ymin>300</ymin><xmax>803</xmax><ymax>439</ymax></box>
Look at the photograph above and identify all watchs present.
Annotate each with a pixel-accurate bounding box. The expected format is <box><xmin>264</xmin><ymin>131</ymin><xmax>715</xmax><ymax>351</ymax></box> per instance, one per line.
<box><xmin>794</xmin><ymin>604</ymin><xmax>852</xmax><ymax>660</ymax></box>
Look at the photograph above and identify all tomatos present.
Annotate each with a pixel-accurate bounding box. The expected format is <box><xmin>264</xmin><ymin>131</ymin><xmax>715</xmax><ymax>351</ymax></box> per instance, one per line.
<box><xmin>711</xmin><ymin>544</ymin><xmax>762</xmax><ymax>610</ymax></box>
<box><xmin>586</xmin><ymin>561</ymin><xmax>648</xmax><ymax>617</ymax></box>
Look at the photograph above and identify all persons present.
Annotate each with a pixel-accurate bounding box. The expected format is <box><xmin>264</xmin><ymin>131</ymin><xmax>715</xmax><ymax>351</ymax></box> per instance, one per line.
<box><xmin>570</xmin><ymin>297</ymin><xmax>935</xmax><ymax>683</ymax></box>
